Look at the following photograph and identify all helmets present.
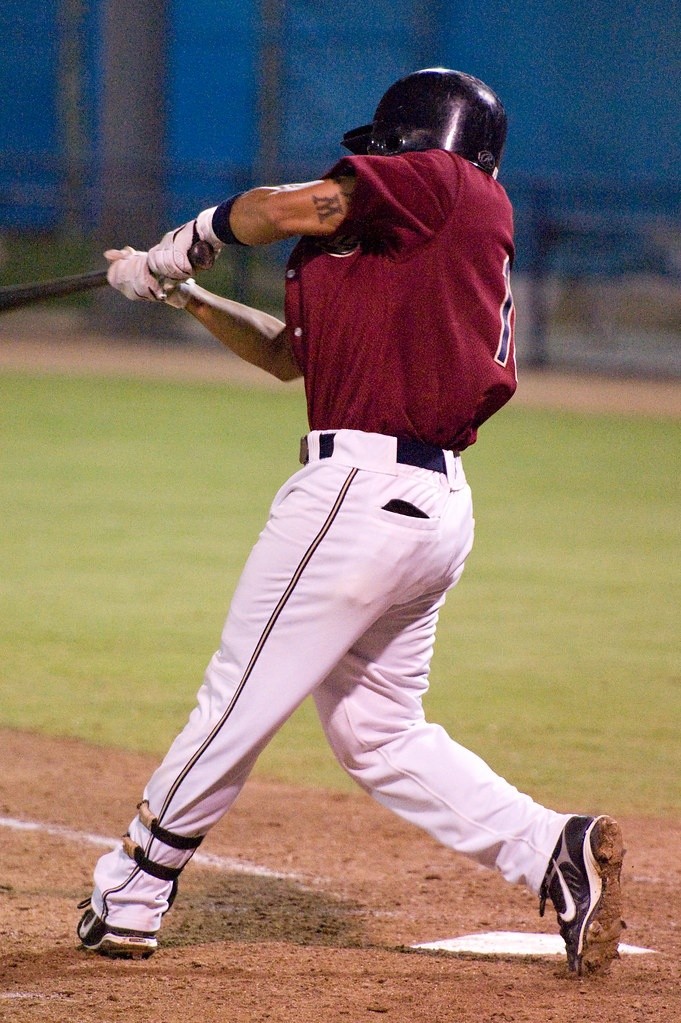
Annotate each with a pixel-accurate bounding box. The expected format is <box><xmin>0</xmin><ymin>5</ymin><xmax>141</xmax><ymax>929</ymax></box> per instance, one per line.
<box><xmin>343</xmin><ymin>66</ymin><xmax>508</xmax><ymax>183</ymax></box>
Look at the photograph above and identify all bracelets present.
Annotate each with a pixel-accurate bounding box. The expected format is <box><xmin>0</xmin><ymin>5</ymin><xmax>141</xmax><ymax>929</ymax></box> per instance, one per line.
<box><xmin>212</xmin><ymin>193</ymin><xmax>248</xmax><ymax>248</ymax></box>
<box><xmin>195</xmin><ymin>206</ymin><xmax>226</xmax><ymax>247</ymax></box>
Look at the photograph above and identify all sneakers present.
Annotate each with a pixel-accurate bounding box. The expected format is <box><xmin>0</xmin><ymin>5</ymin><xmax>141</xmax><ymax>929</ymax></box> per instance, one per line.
<box><xmin>541</xmin><ymin>814</ymin><xmax>623</xmax><ymax>978</ymax></box>
<box><xmin>76</xmin><ymin>896</ymin><xmax>157</xmax><ymax>958</ymax></box>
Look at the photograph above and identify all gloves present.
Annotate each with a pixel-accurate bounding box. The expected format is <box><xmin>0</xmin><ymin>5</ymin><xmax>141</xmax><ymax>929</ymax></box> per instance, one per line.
<box><xmin>148</xmin><ymin>205</ymin><xmax>229</xmax><ymax>282</ymax></box>
<box><xmin>102</xmin><ymin>245</ymin><xmax>195</xmax><ymax>310</ymax></box>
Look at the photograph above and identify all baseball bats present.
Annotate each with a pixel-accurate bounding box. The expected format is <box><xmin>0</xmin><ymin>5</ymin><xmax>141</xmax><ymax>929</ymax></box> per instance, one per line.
<box><xmin>1</xmin><ymin>239</ymin><xmax>217</xmax><ymax>314</ymax></box>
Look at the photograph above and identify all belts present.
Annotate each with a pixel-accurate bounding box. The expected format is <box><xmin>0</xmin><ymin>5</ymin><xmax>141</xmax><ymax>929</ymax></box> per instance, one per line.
<box><xmin>300</xmin><ymin>434</ymin><xmax>458</xmax><ymax>474</ymax></box>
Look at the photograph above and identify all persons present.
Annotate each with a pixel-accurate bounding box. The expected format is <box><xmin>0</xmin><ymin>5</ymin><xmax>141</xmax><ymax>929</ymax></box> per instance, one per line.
<box><xmin>75</xmin><ymin>68</ymin><xmax>626</xmax><ymax>974</ymax></box>
